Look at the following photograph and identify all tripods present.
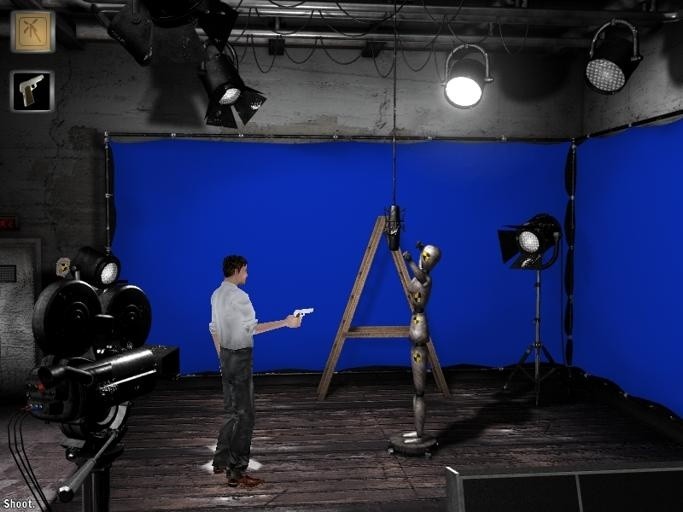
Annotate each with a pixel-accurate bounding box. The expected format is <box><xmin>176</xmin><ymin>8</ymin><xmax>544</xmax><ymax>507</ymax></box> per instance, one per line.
<box><xmin>504</xmin><ymin>267</ymin><xmax>570</xmax><ymax>407</ymax></box>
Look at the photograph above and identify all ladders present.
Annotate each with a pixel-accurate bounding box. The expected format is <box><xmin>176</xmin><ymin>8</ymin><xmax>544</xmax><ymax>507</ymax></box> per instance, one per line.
<box><xmin>317</xmin><ymin>217</ymin><xmax>451</xmax><ymax>401</ymax></box>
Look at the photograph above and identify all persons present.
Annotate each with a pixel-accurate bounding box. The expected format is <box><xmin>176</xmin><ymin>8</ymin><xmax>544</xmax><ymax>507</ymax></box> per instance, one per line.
<box><xmin>205</xmin><ymin>254</ymin><xmax>305</xmax><ymax>488</ymax></box>
